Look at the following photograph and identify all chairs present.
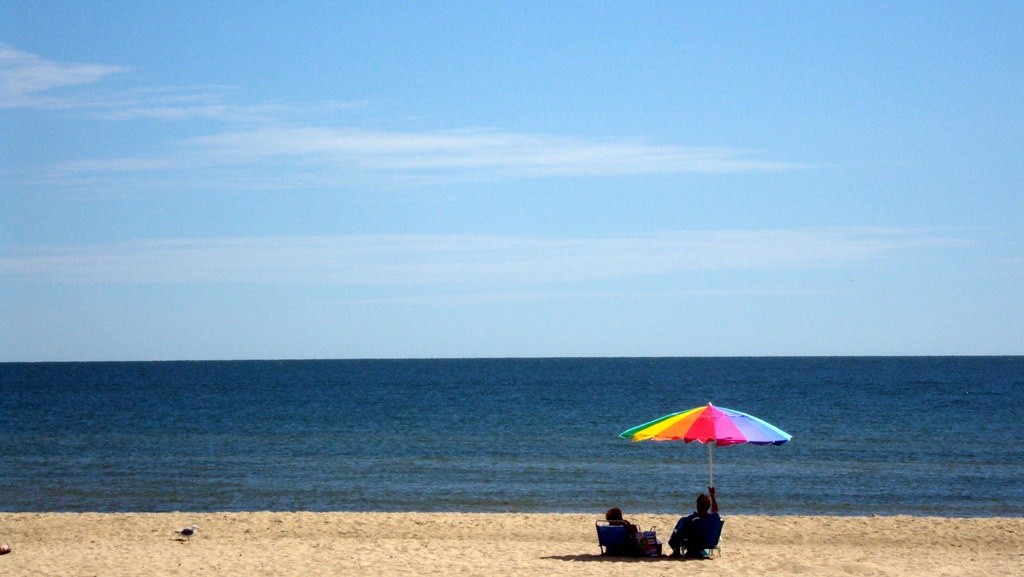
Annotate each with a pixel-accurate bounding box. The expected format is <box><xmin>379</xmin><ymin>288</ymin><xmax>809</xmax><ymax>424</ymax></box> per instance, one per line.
<box><xmin>673</xmin><ymin>514</ymin><xmax>724</xmax><ymax>560</ymax></box>
<box><xmin>595</xmin><ymin>519</ymin><xmax>643</xmax><ymax>557</ymax></box>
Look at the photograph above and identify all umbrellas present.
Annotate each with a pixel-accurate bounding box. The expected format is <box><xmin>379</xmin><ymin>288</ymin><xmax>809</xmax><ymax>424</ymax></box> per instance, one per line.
<box><xmin>617</xmin><ymin>402</ymin><xmax>794</xmax><ymax>510</ymax></box>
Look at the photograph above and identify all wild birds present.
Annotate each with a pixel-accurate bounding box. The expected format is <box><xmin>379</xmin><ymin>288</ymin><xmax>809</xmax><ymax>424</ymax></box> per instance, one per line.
<box><xmin>174</xmin><ymin>525</ymin><xmax>199</xmax><ymax>540</ymax></box>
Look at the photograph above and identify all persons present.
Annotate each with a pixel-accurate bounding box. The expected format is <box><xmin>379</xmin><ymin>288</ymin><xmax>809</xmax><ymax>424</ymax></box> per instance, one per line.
<box><xmin>667</xmin><ymin>486</ymin><xmax>718</xmax><ymax>558</ymax></box>
<box><xmin>605</xmin><ymin>507</ymin><xmax>639</xmax><ymax>556</ymax></box>
